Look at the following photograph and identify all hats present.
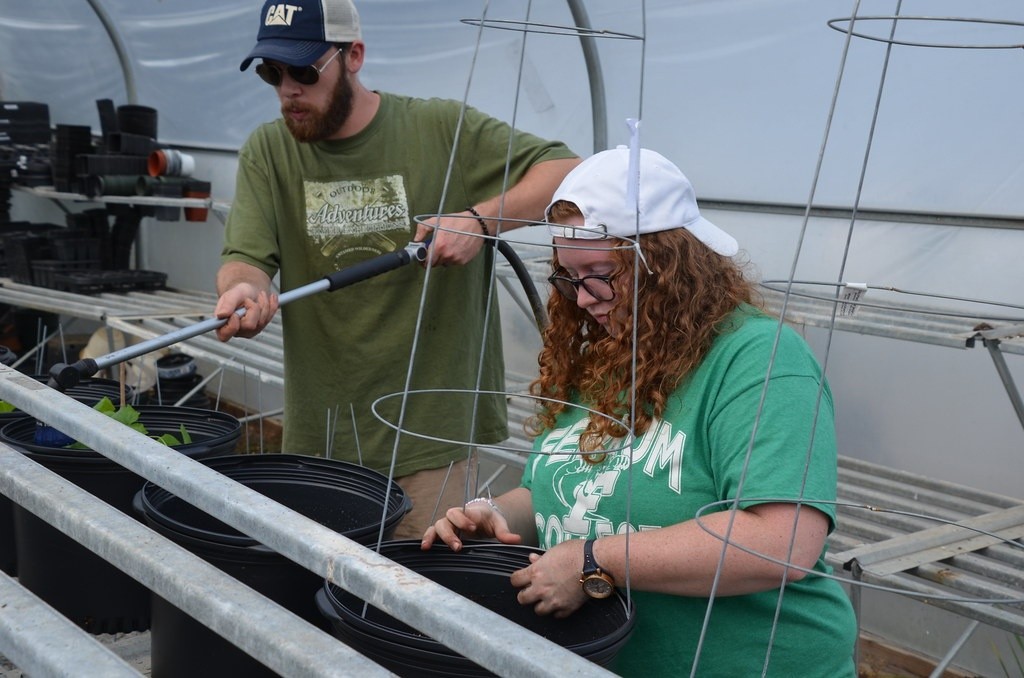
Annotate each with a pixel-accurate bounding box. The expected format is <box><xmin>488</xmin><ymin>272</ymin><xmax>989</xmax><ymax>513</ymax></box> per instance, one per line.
<box><xmin>544</xmin><ymin>145</ymin><xmax>739</xmax><ymax>257</ymax></box>
<box><xmin>240</xmin><ymin>0</ymin><xmax>361</xmax><ymax>71</ymax></box>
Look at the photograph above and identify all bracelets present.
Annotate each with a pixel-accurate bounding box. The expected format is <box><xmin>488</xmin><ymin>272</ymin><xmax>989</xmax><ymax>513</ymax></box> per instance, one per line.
<box><xmin>466</xmin><ymin>497</ymin><xmax>495</xmax><ymax>509</ymax></box>
<box><xmin>464</xmin><ymin>206</ymin><xmax>490</xmax><ymax>244</ymax></box>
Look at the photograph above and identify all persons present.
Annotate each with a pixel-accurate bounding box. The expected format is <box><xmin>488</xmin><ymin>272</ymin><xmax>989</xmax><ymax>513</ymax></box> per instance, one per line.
<box><xmin>214</xmin><ymin>0</ymin><xmax>584</xmax><ymax>479</ymax></box>
<box><xmin>422</xmin><ymin>145</ymin><xmax>859</xmax><ymax>678</ymax></box>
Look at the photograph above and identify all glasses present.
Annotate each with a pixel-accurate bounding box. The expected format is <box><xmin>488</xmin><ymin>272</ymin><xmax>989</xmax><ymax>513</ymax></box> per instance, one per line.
<box><xmin>548</xmin><ymin>261</ymin><xmax>632</xmax><ymax>302</ymax></box>
<box><xmin>256</xmin><ymin>47</ymin><xmax>343</xmax><ymax>86</ymax></box>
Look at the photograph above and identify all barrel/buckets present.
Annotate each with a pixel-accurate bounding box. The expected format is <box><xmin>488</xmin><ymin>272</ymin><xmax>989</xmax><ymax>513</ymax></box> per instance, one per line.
<box><xmin>133</xmin><ymin>453</ymin><xmax>413</xmax><ymax>678</ymax></box>
<box><xmin>316</xmin><ymin>538</ymin><xmax>637</xmax><ymax>678</ymax></box>
<box><xmin>0</xmin><ymin>374</ymin><xmax>242</xmax><ymax>636</ymax></box>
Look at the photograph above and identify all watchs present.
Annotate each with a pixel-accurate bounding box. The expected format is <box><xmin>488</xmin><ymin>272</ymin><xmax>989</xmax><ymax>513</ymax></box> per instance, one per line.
<box><xmin>580</xmin><ymin>538</ymin><xmax>616</xmax><ymax>599</ymax></box>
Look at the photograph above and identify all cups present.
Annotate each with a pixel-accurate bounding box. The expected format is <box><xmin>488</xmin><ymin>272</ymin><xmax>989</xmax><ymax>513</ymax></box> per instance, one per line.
<box><xmin>79</xmin><ymin>325</ymin><xmax>126</xmax><ymax>370</ymax></box>
<box><xmin>118</xmin><ymin>102</ymin><xmax>158</xmax><ymax>138</ymax></box>
<box><xmin>139</xmin><ymin>174</ymin><xmax>201</xmax><ymax>193</ymax></box>
<box><xmin>146</xmin><ymin>148</ymin><xmax>196</xmax><ymax>177</ymax></box>
<box><xmin>52</xmin><ymin>123</ymin><xmax>92</xmax><ymax>191</ymax></box>
<box><xmin>153</xmin><ymin>180</ymin><xmax>182</xmax><ymax>221</ymax></box>
<box><xmin>184</xmin><ymin>180</ymin><xmax>210</xmax><ymax>221</ymax></box>
<box><xmin>95</xmin><ymin>175</ymin><xmax>139</xmax><ymax>195</ymax></box>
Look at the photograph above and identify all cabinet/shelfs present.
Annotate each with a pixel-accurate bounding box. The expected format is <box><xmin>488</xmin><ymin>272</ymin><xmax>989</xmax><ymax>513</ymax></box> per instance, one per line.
<box><xmin>0</xmin><ymin>174</ymin><xmax>1024</xmax><ymax>636</ymax></box>
